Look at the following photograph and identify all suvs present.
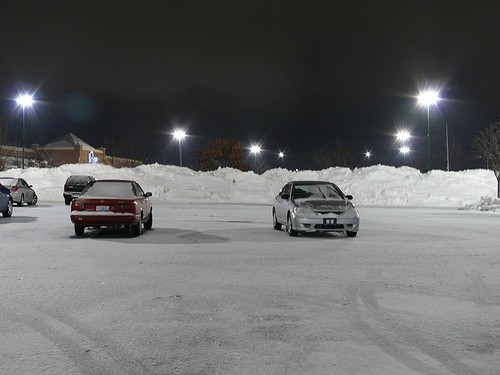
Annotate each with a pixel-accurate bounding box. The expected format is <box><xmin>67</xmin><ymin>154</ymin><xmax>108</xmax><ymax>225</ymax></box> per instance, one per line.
<box><xmin>63</xmin><ymin>175</ymin><xmax>97</xmax><ymax>205</ymax></box>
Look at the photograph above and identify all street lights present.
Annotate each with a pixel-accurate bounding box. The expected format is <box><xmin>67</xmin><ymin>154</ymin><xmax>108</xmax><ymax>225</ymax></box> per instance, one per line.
<box><xmin>251</xmin><ymin>145</ymin><xmax>260</xmax><ymax>174</ymax></box>
<box><xmin>398</xmin><ymin>132</ymin><xmax>410</xmax><ymax>166</ymax></box>
<box><xmin>18</xmin><ymin>93</ymin><xmax>33</xmax><ymax>169</ymax></box>
<box><xmin>422</xmin><ymin>91</ymin><xmax>434</xmax><ymax>171</ymax></box>
<box><xmin>175</xmin><ymin>130</ymin><xmax>185</xmax><ymax>167</ymax></box>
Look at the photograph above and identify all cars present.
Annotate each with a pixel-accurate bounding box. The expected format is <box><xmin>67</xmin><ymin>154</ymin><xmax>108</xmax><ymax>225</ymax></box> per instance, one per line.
<box><xmin>0</xmin><ymin>183</ymin><xmax>13</xmax><ymax>217</ymax></box>
<box><xmin>0</xmin><ymin>177</ymin><xmax>38</xmax><ymax>207</ymax></box>
<box><xmin>271</xmin><ymin>181</ymin><xmax>360</xmax><ymax>238</ymax></box>
<box><xmin>70</xmin><ymin>179</ymin><xmax>153</xmax><ymax>238</ymax></box>
